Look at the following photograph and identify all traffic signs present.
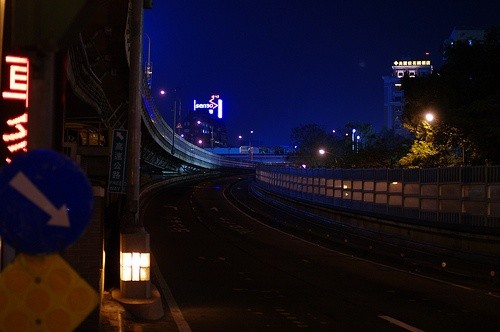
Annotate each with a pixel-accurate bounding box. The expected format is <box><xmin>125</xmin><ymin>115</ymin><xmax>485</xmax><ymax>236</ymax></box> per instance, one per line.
<box><xmin>107</xmin><ymin>128</ymin><xmax>128</xmax><ymax>194</ymax></box>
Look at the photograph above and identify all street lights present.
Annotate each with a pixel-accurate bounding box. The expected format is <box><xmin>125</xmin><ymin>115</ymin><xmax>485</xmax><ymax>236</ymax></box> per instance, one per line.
<box><xmin>319</xmin><ymin>149</ymin><xmax>344</xmax><ymax>169</ymax></box>
